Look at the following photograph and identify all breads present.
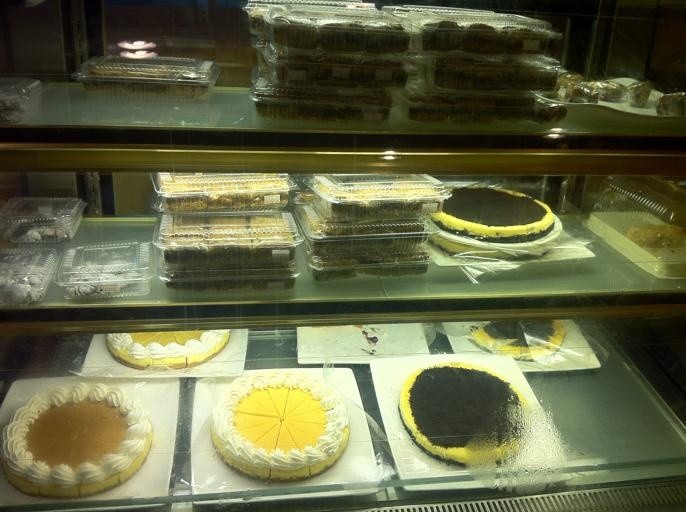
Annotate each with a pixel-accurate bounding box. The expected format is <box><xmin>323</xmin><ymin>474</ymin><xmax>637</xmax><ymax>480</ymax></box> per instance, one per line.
<box><xmin>543</xmin><ymin>71</ymin><xmax>685</xmax><ymax>116</ymax></box>
<box><xmin>158</xmin><ymin>172</ymin><xmax>300</xmax><ymax>289</ymax></box>
<box><xmin>402</xmin><ymin>10</ymin><xmax>565</xmax><ymax>124</ymax></box>
<box><xmin>627</xmin><ymin>222</ymin><xmax>683</xmax><ymax>248</ymax></box>
<box><xmin>296</xmin><ymin>173</ymin><xmax>443</xmax><ymax>282</ymax></box>
<box><xmin>250</xmin><ymin>8</ymin><xmax>409</xmax><ymax>124</ymax></box>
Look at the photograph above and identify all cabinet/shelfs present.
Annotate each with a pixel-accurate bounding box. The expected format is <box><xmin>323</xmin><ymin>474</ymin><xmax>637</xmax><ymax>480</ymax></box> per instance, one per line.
<box><xmin>0</xmin><ymin>76</ymin><xmax>686</xmax><ymax>511</ymax></box>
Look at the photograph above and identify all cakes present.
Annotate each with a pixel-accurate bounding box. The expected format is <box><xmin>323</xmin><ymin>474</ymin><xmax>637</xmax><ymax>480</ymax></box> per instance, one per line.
<box><xmin>398</xmin><ymin>361</ymin><xmax>531</xmax><ymax>468</ymax></box>
<box><xmin>1</xmin><ymin>382</ymin><xmax>154</xmax><ymax>500</ymax></box>
<box><xmin>312</xmin><ymin>324</ymin><xmax>395</xmax><ymax>356</ymax></box>
<box><xmin>104</xmin><ymin>329</ymin><xmax>231</xmax><ymax>372</ymax></box>
<box><xmin>81</xmin><ymin>61</ymin><xmax>210</xmax><ymax>99</ymax></box>
<box><xmin>429</xmin><ymin>188</ymin><xmax>563</xmax><ymax>262</ymax></box>
<box><xmin>209</xmin><ymin>371</ymin><xmax>351</xmax><ymax>482</ymax></box>
<box><xmin>469</xmin><ymin>318</ymin><xmax>566</xmax><ymax>361</ymax></box>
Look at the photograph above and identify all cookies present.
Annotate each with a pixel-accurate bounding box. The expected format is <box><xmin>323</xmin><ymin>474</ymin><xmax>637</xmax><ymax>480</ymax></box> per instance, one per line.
<box><xmin>0</xmin><ymin>212</ymin><xmax>138</xmax><ymax>304</ymax></box>
